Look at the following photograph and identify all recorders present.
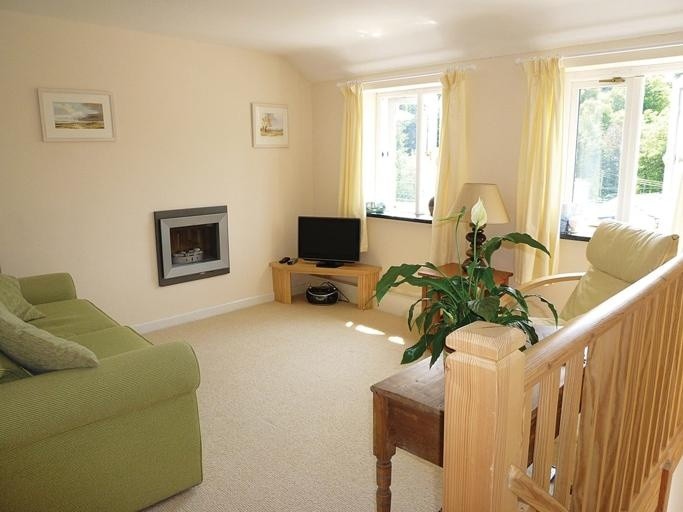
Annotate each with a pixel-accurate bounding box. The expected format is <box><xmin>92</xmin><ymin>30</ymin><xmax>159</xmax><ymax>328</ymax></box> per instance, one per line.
<box><xmin>306</xmin><ymin>286</ymin><xmax>338</xmax><ymax>305</ymax></box>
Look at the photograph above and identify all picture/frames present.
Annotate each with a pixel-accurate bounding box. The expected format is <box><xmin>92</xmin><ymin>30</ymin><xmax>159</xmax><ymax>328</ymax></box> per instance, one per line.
<box><xmin>37</xmin><ymin>86</ymin><xmax>117</xmax><ymax>143</ymax></box>
<box><xmin>251</xmin><ymin>102</ymin><xmax>289</xmax><ymax>148</ymax></box>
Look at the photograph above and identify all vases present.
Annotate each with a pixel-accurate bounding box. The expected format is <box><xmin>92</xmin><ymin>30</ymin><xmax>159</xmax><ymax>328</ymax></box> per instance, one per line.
<box><xmin>441</xmin><ymin>346</ymin><xmax>456</xmax><ymax>373</ymax></box>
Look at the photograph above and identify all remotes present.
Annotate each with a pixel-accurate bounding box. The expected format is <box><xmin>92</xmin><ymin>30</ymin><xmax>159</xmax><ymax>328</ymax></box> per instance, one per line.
<box><xmin>279</xmin><ymin>256</ymin><xmax>290</xmax><ymax>264</ymax></box>
<box><xmin>287</xmin><ymin>258</ymin><xmax>297</xmax><ymax>265</ymax></box>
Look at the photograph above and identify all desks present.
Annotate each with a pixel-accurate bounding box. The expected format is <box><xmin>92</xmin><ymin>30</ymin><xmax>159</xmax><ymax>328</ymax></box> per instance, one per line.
<box><xmin>369</xmin><ymin>325</ymin><xmax>588</xmax><ymax>511</ymax></box>
<box><xmin>269</xmin><ymin>258</ymin><xmax>381</xmax><ymax>310</ymax></box>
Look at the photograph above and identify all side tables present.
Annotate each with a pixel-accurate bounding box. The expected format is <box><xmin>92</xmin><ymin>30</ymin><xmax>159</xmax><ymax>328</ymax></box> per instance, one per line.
<box><xmin>418</xmin><ymin>262</ymin><xmax>513</xmax><ymax>354</ymax></box>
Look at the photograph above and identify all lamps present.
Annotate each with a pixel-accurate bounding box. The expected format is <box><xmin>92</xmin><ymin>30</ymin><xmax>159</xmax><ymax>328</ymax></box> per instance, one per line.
<box><xmin>449</xmin><ymin>183</ymin><xmax>509</xmax><ymax>269</ymax></box>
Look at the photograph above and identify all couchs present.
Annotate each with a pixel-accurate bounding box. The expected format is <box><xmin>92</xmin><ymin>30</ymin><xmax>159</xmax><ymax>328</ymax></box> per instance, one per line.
<box><xmin>0</xmin><ymin>273</ymin><xmax>204</xmax><ymax>512</ymax></box>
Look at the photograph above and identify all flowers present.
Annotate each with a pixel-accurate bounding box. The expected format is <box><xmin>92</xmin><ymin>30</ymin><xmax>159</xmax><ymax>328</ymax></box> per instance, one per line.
<box><xmin>377</xmin><ymin>199</ymin><xmax>559</xmax><ymax>370</ymax></box>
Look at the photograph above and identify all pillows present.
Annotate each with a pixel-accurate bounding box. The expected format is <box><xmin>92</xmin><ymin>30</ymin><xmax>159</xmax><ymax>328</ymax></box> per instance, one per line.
<box><xmin>0</xmin><ymin>273</ymin><xmax>45</xmax><ymax>321</ymax></box>
<box><xmin>0</xmin><ymin>300</ymin><xmax>99</xmax><ymax>371</ymax></box>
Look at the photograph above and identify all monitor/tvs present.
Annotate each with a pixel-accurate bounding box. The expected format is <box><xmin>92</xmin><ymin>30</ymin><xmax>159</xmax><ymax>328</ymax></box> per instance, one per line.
<box><xmin>298</xmin><ymin>216</ymin><xmax>361</xmax><ymax>269</ymax></box>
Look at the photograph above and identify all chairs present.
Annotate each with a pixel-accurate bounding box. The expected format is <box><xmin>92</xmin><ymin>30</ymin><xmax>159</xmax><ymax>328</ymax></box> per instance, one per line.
<box><xmin>504</xmin><ymin>221</ymin><xmax>680</xmax><ymax>365</ymax></box>
